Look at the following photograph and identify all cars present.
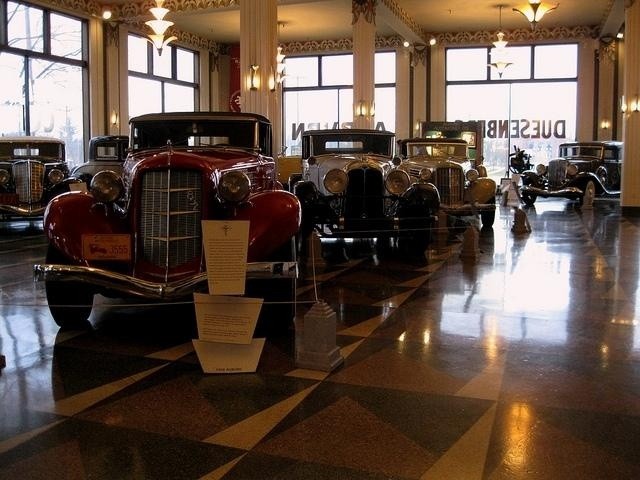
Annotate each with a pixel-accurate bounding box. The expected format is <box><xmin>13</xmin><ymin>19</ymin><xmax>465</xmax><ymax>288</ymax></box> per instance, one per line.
<box><xmin>519</xmin><ymin>140</ymin><xmax>623</xmax><ymax>205</ymax></box>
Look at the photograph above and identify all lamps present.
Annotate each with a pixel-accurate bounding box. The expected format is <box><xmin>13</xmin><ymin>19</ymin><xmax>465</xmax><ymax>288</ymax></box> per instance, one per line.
<box><xmin>139</xmin><ymin>1</ymin><xmax>179</xmax><ymax>60</ymax></box>
<box><xmin>510</xmin><ymin>1</ymin><xmax>559</xmax><ymax>30</ymax></box>
<box><xmin>352</xmin><ymin>96</ymin><xmax>379</xmax><ymax>123</ymax></box>
<box><xmin>483</xmin><ymin>4</ymin><xmax>514</xmax><ymax>80</ymax></box>
<box><xmin>245</xmin><ymin>41</ymin><xmax>292</xmax><ymax>97</ymax></box>
<box><xmin>599</xmin><ymin>94</ymin><xmax>639</xmax><ymax>130</ymax></box>
